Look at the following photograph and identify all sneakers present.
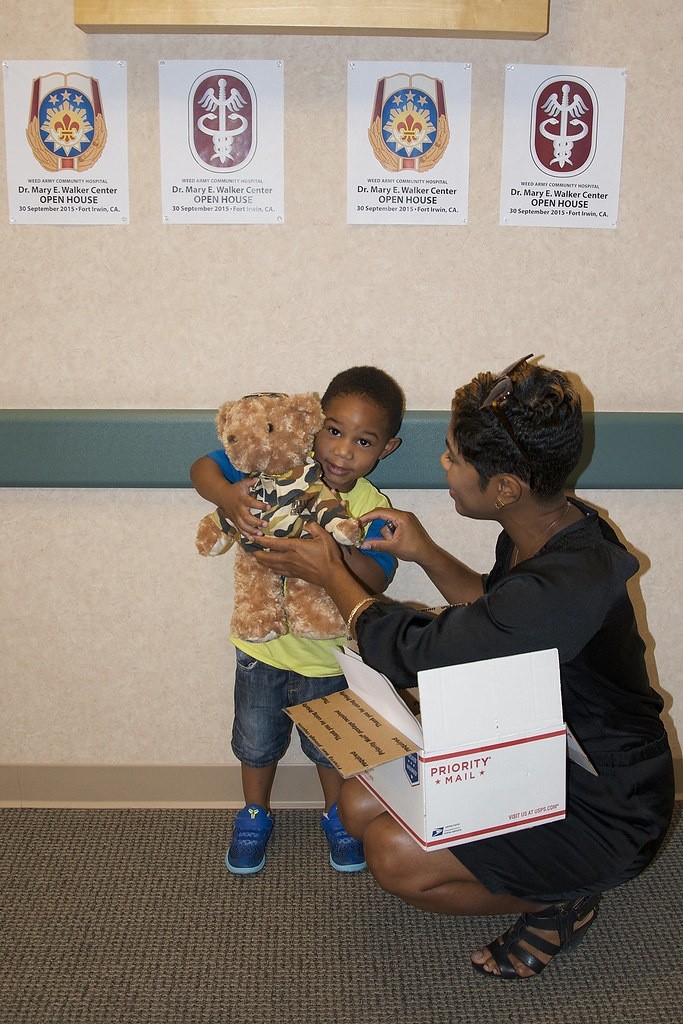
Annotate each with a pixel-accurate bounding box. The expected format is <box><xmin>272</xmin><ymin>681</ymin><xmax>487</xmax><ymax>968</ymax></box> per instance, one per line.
<box><xmin>225</xmin><ymin>804</ymin><xmax>275</xmax><ymax>874</ymax></box>
<box><xmin>321</xmin><ymin>802</ymin><xmax>368</xmax><ymax>872</ymax></box>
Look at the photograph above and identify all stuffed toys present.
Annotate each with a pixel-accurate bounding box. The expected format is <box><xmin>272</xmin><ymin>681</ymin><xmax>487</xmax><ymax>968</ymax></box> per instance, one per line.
<box><xmin>194</xmin><ymin>390</ymin><xmax>362</xmax><ymax>646</ymax></box>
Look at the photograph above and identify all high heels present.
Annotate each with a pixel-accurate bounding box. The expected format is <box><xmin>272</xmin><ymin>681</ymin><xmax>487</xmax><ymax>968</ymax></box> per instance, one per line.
<box><xmin>469</xmin><ymin>894</ymin><xmax>599</xmax><ymax>980</ymax></box>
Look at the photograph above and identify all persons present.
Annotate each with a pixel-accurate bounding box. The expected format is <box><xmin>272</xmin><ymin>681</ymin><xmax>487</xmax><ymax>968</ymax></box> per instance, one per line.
<box><xmin>188</xmin><ymin>364</ymin><xmax>407</xmax><ymax>875</ymax></box>
<box><xmin>248</xmin><ymin>353</ymin><xmax>677</xmax><ymax>981</ymax></box>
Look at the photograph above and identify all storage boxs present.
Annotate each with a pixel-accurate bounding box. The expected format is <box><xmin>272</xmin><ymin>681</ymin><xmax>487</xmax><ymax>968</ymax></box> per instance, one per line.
<box><xmin>281</xmin><ymin>647</ymin><xmax>601</xmax><ymax>854</ymax></box>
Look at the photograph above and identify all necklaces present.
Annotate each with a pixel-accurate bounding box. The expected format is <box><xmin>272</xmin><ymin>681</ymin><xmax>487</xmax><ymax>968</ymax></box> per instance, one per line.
<box><xmin>513</xmin><ymin>501</ymin><xmax>571</xmax><ymax>565</ymax></box>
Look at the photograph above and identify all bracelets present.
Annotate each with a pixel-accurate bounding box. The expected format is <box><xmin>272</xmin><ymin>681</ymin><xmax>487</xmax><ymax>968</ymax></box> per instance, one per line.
<box><xmin>346</xmin><ymin>597</ymin><xmax>381</xmax><ymax>639</ymax></box>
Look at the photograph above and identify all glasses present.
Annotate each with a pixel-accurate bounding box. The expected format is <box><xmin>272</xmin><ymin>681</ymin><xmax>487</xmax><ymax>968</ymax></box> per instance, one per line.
<box><xmin>479</xmin><ymin>354</ymin><xmax>538</xmax><ymax>490</ymax></box>
